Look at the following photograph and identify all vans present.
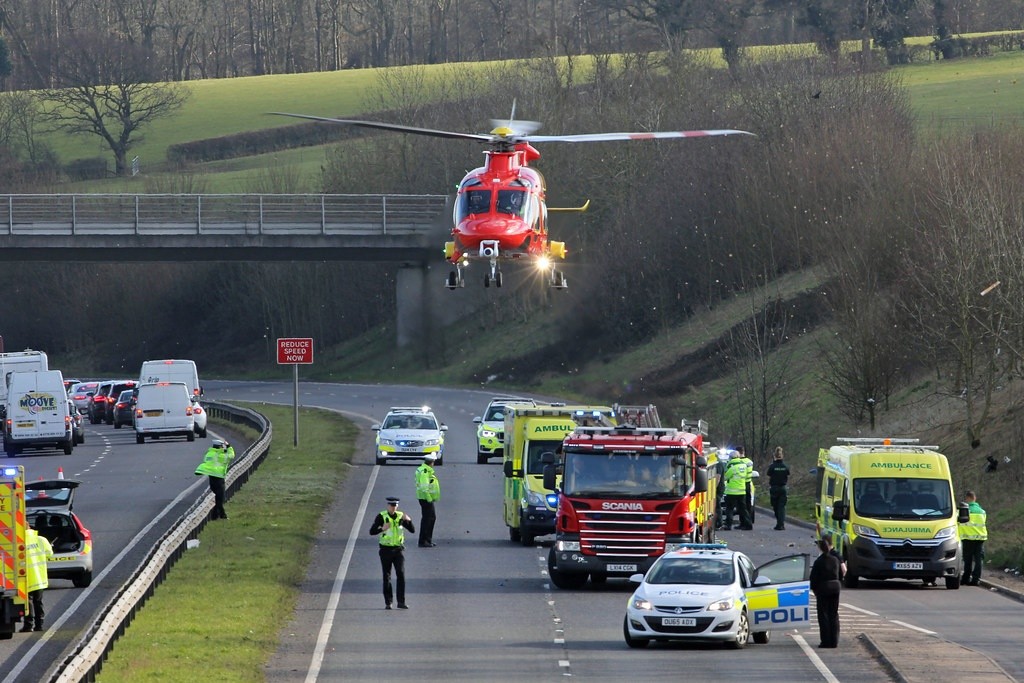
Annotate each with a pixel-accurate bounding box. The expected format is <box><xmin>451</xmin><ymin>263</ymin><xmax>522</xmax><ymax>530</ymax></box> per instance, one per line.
<box><xmin>0</xmin><ymin>370</ymin><xmax>72</xmax><ymax>458</ymax></box>
<box><xmin>134</xmin><ymin>360</ymin><xmax>203</xmax><ymax>444</ymax></box>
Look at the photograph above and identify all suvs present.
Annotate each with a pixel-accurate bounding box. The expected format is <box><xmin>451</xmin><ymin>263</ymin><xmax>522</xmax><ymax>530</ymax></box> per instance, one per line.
<box><xmin>716</xmin><ymin>448</ymin><xmax>760</xmax><ymax>523</ymax></box>
<box><xmin>25</xmin><ymin>478</ymin><xmax>92</xmax><ymax>587</ymax></box>
<box><xmin>473</xmin><ymin>397</ymin><xmax>536</xmax><ymax>464</ymax></box>
<box><xmin>64</xmin><ymin>379</ymin><xmax>140</xmax><ymax>429</ymax></box>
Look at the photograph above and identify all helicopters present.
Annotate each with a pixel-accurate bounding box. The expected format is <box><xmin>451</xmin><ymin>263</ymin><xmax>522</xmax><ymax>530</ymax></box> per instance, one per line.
<box><xmin>263</xmin><ymin>100</ymin><xmax>759</xmax><ymax>291</ymax></box>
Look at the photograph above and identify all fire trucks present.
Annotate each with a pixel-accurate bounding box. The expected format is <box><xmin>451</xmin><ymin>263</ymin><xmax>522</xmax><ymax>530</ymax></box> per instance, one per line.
<box><xmin>541</xmin><ymin>403</ymin><xmax>719</xmax><ymax>589</ymax></box>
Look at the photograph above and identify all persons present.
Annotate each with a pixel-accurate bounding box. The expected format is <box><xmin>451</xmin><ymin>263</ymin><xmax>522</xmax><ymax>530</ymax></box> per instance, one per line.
<box><xmin>468</xmin><ymin>192</ymin><xmax>482</xmax><ymax>212</ymax></box>
<box><xmin>507</xmin><ymin>193</ymin><xmax>522</xmax><ymax>212</ymax></box>
<box><xmin>809</xmin><ymin>540</ymin><xmax>839</xmax><ymax>648</ymax></box>
<box><xmin>195</xmin><ymin>440</ymin><xmax>235</xmax><ymax>520</ymax></box>
<box><xmin>735</xmin><ymin>446</ymin><xmax>752</xmax><ymax>530</ymax></box>
<box><xmin>369</xmin><ymin>496</ymin><xmax>415</xmax><ymax>610</ymax></box>
<box><xmin>958</xmin><ymin>493</ymin><xmax>987</xmax><ymax>586</ymax></box>
<box><xmin>722</xmin><ymin>450</ymin><xmax>748</xmax><ymax>530</ymax></box>
<box><xmin>822</xmin><ymin>534</ymin><xmax>848</xmax><ymax>581</ymax></box>
<box><xmin>415</xmin><ymin>456</ymin><xmax>439</xmax><ymax>547</ymax></box>
<box><xmin>19</xmin><ymin>522</ymin><xmax>53</xmax><ymax>631</ymax></box>
<box><xmin>767</xmin><ymin>447</ymin><xmax>789</xmax><ymax>530</ymax></box>
<box><xmin>573</xmin><ymin>456</ymin><xmax>588</xmax><ymax>486</ymax></box>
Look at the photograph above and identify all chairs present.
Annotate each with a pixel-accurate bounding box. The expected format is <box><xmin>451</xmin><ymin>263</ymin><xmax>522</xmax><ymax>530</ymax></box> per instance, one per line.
<box><xmin>391</xmin><ymin>419</ymin><xmax>405</xmax><ymax>427</ymax></box>
<box><xmin>532</xmin><ymin>448</ymin><xmax>548</xmax><ymax>474</ymax></box>
<box><xmin>664</xmin><ymin>564</ymin><xmax>693</xmax><ymax>584</ymax></box>
<box><xmin>717</xmin><ymin>562</ymin><xmax>731</xmax><ymax>579</ymax></box>
<box><xmin>858</xmin><ymin>481</ymin><xmax>940</xmax><ymax>513</ymax></box>
<box><xmin>35</xmin><ymin>515</ymin><xmax>47</xmax><ymax>527</ymax></box>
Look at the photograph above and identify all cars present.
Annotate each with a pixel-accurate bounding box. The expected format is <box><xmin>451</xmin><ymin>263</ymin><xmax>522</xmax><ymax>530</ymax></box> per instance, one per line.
<box><xmin>113</xmin><ymin>389</ymin><xmax>139</xmax><ymax>429</ymax></box>
<box><xmin>623</xmin><ymin>544</ymin><xmax>810</xmax><ymax>648</ymax></box>
<box><xmin>55</xmin><ymin>399</ymin><xmax>84</xmax><ymax>446</ymax></box>
<box><xmin>190</xmin><ymin>394</ymin><xmax>207</xmax><ymax>437</ymax></box>
<box><xmin>372</xmin><ymin>407</ymin><xmax>448</xmax><ymax>466</ymax></box>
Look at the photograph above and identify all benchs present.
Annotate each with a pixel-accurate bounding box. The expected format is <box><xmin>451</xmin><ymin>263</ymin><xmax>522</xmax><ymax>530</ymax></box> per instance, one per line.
<box><xmin>31</xmin><ymin>515</ymin><xmax>75</xmax><ymax>552</ymax></box>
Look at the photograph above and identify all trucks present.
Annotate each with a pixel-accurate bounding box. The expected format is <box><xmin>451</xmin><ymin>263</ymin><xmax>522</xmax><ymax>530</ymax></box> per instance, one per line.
<box><xmin>0</xmin><ymin>349</ymin><xmax>48</xmax><ymax>427</ymax></box>
<box><xmin>0</xmin><ymin>465</ymin><xmax>29</xmax><ymax>639</ymax></box>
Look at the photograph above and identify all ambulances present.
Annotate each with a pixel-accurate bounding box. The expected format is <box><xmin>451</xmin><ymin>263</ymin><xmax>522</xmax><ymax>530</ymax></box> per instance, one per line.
<box><xmin>502</xmin><ymin>402</ymin><xmax>618</xmax><ymax>546</ymax></box>
<box><xmin>809</xmin><ymin>437</ymin><xmax>969</xmax><ymax>589</ymax></box>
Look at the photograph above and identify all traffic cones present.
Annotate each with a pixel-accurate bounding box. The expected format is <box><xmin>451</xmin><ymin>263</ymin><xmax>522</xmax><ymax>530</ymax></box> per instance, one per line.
<box><xmin>35</xmin><ymin>476</ymin><xmax>49</xmax><ymax>497</ymax></box>
<box><xmin>57</xmin><ymin>467</ymin><xmax>67</xmax><ymax>490</ymax></box>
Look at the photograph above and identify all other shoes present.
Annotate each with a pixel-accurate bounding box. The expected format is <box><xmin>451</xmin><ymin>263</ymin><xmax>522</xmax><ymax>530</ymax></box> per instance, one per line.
<box><xmin>734</xmin><ymin>525</ymin><xmax>741</xmax><ymax>529</ymax></box>
<box><xmin>431</xmin><ymin>541</ymin><xmax>437</xmax><ymax>546</ymax></box>
<box><xmin>385</xmin><ymin>605</ymin><xmax>393</xmax><ymax>610</ymax></box>
<box><xmin>774</xmin><ymin>525</ymin><xmax>785</xmax><ymax>530</ymax></box>
<box><xmin>398</xmin><ymin>604</ymin><xmax>409</xmax><ymax>609</ymax></box>
<box><xmin>961</xmin><ymin>580</ymin><xmax>971</xmax><ymax>586</ymax></box>
<box><xmin>724</xmin><ymin>526</ymin><xmax>731</xmax><ymax>531</ymax></box>
<box><xmin>971</xmin><ymin>580</ymin><xmax>980</xmax><ymax>587</ymax></box>
<box><xmin>219</xmin><ymin>513</ymin><xmax>227</xmax><ymax>519</ymax></box>
<box><xmin>418</xmin><ymin>542</ymin><xmax>433</xmax><ymax>547</ymax></box>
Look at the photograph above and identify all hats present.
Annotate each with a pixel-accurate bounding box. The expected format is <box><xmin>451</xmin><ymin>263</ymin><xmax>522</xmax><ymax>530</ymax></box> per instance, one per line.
<box><xmin>815</xmin><ymin>540</ymin><xmax>829</xmax><ymax>552</ymax></box>
<box><xmin>386</xmin><ymin>496</ymin><xmax>400</xmax><ymax>507</ymax></box>
<box><xmin>212</xmin><ymin>439</ymin><xmax>224</xmax><ymax>446</ymax></box>
<box><xmin>424</xmin><ymin>454</ymin><xmax>438</xmax><ymax>461</ymax></box>
<box><xmin>773</xmin><ymin>447</ymin><xmax>783</xmax><ymax>458</ymax></box>
<box><xmin>729</xmin><ymin>451</ymin><xmax>740</xmax><ymax>459</ymax></box>
